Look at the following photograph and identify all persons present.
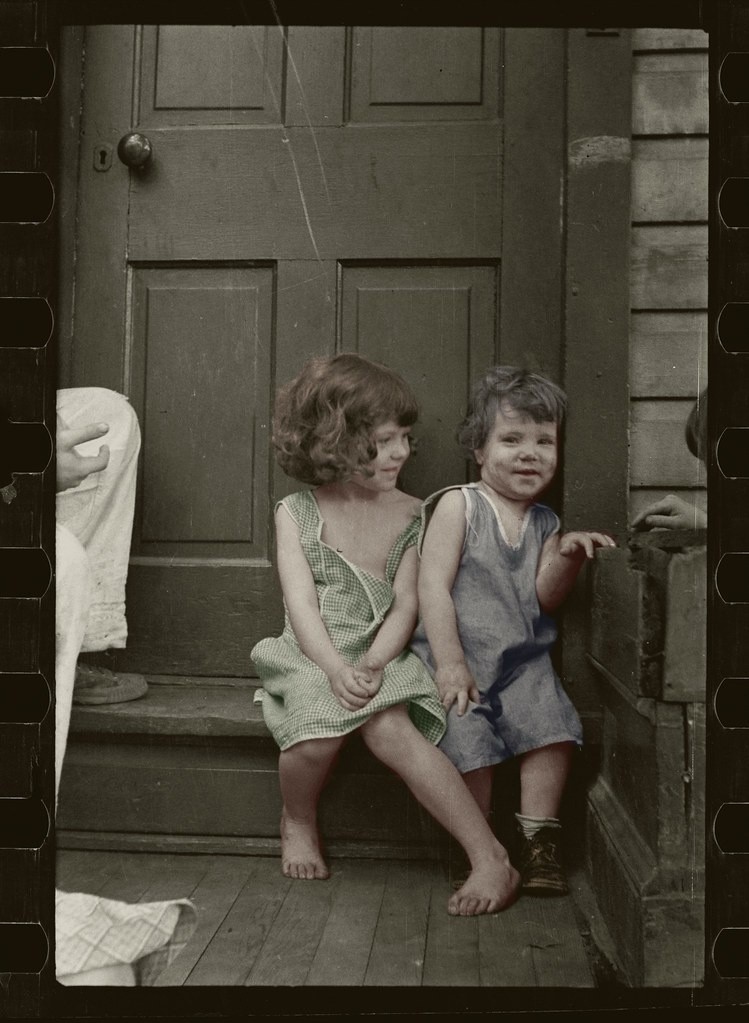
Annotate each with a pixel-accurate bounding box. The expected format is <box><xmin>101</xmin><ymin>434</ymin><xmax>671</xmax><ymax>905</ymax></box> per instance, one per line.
<box><xmin>630</xmin><ymin>389</ymin><xmax>708</xmax><ymax>529</ymax></box>
<box><xmin>409</xmin><ymin>367</ymin><xmax>616</xmax><ymax>893</ymax></box>
<box><xmin>55</xmin><ymin>387</ymin><xmax>142</xmax><ymax>815</ymax></box>
<box><xmin>248</xmin><ymin>354</ymin><xmax>521</xmax><ymax>916</ymax></box>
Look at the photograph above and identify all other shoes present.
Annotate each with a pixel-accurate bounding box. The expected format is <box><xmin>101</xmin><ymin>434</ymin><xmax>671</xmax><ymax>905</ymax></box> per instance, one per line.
<box><xmin>443</xmin><ymin>832</ymin><xmax>473</xmax><ymax>890</ymax></box>
<box><xmin>514</xmin><ymin>818</ymin><xmax>567</xmax><ymax>891</ymax></box>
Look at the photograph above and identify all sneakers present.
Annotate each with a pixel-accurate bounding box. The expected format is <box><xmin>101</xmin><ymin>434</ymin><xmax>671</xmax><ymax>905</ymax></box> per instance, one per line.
<box><xmin>72</xmin><ymin>662</ymin><xmax>148</xmax><ymax>704</ymax></box>
